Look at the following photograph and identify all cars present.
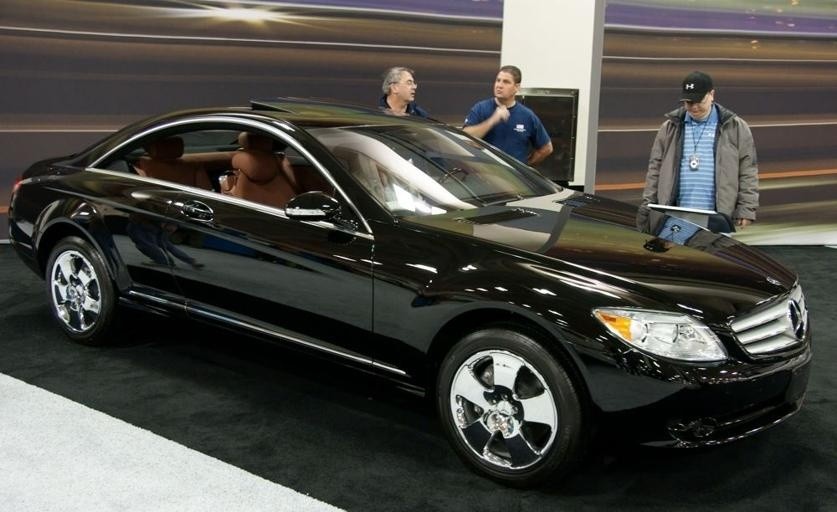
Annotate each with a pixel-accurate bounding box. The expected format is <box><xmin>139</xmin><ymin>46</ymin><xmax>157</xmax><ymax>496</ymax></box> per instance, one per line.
<box><xmin>7</xmin><ymin>96</ymin><xmax>815</xmax><ymax>489</ymax></box>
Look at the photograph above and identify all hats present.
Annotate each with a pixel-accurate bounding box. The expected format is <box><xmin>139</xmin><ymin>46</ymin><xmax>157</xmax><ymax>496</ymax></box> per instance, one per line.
<box><xmin>680</xmin><ymin>70</ymin><xmax>713</xmax><ymax>103</ymax></box>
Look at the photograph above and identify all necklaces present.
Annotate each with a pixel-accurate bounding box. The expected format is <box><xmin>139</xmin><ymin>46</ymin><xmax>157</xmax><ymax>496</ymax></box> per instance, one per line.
<box><xmin>662</xmin><ymin>232</ymin><xmax>674</xmax><ymax>241</ymax></box>
<box><xmin>686</xmin><ymin>111</ymin><xmax>711</xmax><ymax>171</ymax></box>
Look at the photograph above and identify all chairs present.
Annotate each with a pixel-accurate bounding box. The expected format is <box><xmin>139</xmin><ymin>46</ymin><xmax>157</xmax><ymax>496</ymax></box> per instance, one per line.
<box><xmin>138</xmin><ymin>131</ymin><xmax>302</xmax><ymax>210</ymax></box>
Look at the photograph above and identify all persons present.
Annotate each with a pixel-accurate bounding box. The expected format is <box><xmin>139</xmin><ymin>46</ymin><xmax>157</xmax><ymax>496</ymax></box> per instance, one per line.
<box><xmin>635</xmin><ymin>205</ymin><xmax>741</xmax><ymax>257</ymax></box>
<box><xmin>638</xmin><ymin>69</ymin><xmax>759</xmax><ymax>234</ymax></box>
<box><xmin>375</xmin><ymin>66</ymin><xmax>433</xmax><ymax>126</ymax></box>
<box><xmin>458</xmin><ymin>66</ymin><xmax>553</xmax><ymax>170</ymax></box>
